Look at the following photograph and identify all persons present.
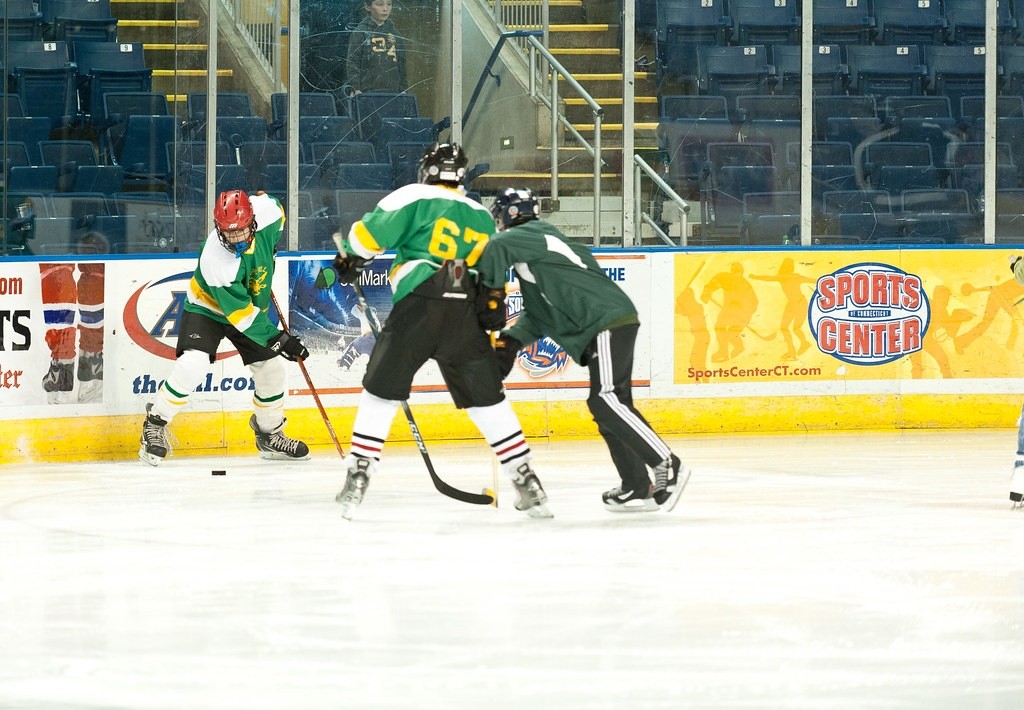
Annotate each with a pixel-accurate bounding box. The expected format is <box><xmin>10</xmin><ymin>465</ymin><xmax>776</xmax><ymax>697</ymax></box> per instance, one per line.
<box><xmin>332</xmin><ymin>142</ymin><xmax>553</xmax><ymax>519</ymax></box>
<box><xmin>139</xmin><ymin>191</ymin><xmax>310</xmax><ymax>464</ymax></box>
<box><xmin>346</xmin><ymin>0</ymin><xmax>408</xmax><ymax>95</ymax></box>
<box><xmin>1010</xmin><ymin>403</ymin><xmax>1024</xmax><ymax>508</ymax></box>
<box><xmin>480</xmin><ymin>187</ymin><xmax>691</xmax><ymax>515</ymax></box>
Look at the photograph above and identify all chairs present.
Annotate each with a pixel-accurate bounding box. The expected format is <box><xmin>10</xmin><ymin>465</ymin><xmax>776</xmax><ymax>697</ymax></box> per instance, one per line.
<box><xmin>626</xmin><ymin>0</ymin><xmax>1024</xmax><ymax>244</ymax></box>
<box><xmin>0</xmin><ymin>0</ymin><xmax>444</xmax><ymax>256</ymax></box>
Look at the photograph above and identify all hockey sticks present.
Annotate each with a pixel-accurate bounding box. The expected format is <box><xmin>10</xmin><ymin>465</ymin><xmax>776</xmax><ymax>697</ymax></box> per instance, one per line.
<box><xmin>333</xmin><ymin>231</ymin><xmax>495</xmax><ymax>506</ymax></box>
<box><xmin>270</xmin><ymin>288</ymin><xmax>381</xmax><ymax>465</ymax></box>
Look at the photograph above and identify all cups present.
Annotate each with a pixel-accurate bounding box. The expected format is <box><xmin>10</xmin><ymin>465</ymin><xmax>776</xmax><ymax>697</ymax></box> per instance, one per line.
<box><xmin>15</xmin><ymin>203</ymin><xmax>33</xmax><ymax>231</ymax></box>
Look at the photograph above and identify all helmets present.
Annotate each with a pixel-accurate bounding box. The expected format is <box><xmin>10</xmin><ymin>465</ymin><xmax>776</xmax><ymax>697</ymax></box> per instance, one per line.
<box><xmin>489</xmin><ymin>186</ymin><xmax>540</xmax><ymax>232</ymax></box>
<box><xmin>417</xmin><ymin>142</ymin><xmax>468</xmax><ymax>189</ymax></box>
<box><xmin>5</xmin><ymin>197</ymin><xmax>37</xmax><ymax>240</ymax></box>
<box><xmin>213</xmin><ymin>189</ymin><xmax>258</xmax><ymax>255</ymax></box>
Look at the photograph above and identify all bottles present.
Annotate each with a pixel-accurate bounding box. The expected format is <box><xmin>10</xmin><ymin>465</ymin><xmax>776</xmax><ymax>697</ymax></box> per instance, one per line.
<box><xmin>782</xmin><ymin>234</ymin><xmax>792</xmax><ymax>245</ymax></box>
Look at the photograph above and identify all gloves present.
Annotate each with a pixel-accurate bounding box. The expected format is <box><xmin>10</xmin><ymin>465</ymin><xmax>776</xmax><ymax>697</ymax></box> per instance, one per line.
<box><xmin>266</xmin><ymin>329</ymin><xmax>309</xmax><ymax>362</ymax></box>
<box><xmin>333</xmin><ymin>252</ymin><xmax>374</xmax><ymax>283</ymax></box>
<box><xmin>477</xmin><ymin>285</ymin><xmax>507</xmax><ymax>331</ymax></box>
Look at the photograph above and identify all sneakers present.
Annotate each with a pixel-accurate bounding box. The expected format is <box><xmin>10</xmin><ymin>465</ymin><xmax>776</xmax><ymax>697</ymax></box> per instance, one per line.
<box><xmin>335</xmin><ymin>454</ymin><xmax>374</xmax><ymax>507</ymax></box>
<box><xmin>652</xmin><ymin>453</ymin><xmax>688</xmax><ymax>505</ymax></box>
<box><xmin>249</xmin><ymin>414</ymin><xmax>310</xmax><ymax>458</ymax></box>
<box><xmin>602</xmin><ymin>485</ymin><xmax>658</xmax><ymax>507</ymax></box>
<box><xmin>1009</xmin><ymin>467</ymin><xmax>1024</xmax><ymax>502</ymax></box>
<box><xmin>508</xmin><ymin>456</ymin><xmax>549</xmax><ymax>511</ymax></box>
<box><xmin>139</xmin><ymin>402</ymin><xmax>172</xmax><ymax>460</ymax></box>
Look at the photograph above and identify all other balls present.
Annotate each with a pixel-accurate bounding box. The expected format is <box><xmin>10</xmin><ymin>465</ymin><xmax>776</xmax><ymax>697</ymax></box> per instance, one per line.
<box><xmin>212</xmin><ymin>470</ymin><xmax>226</xmax><ymax>475</ymax></box>
